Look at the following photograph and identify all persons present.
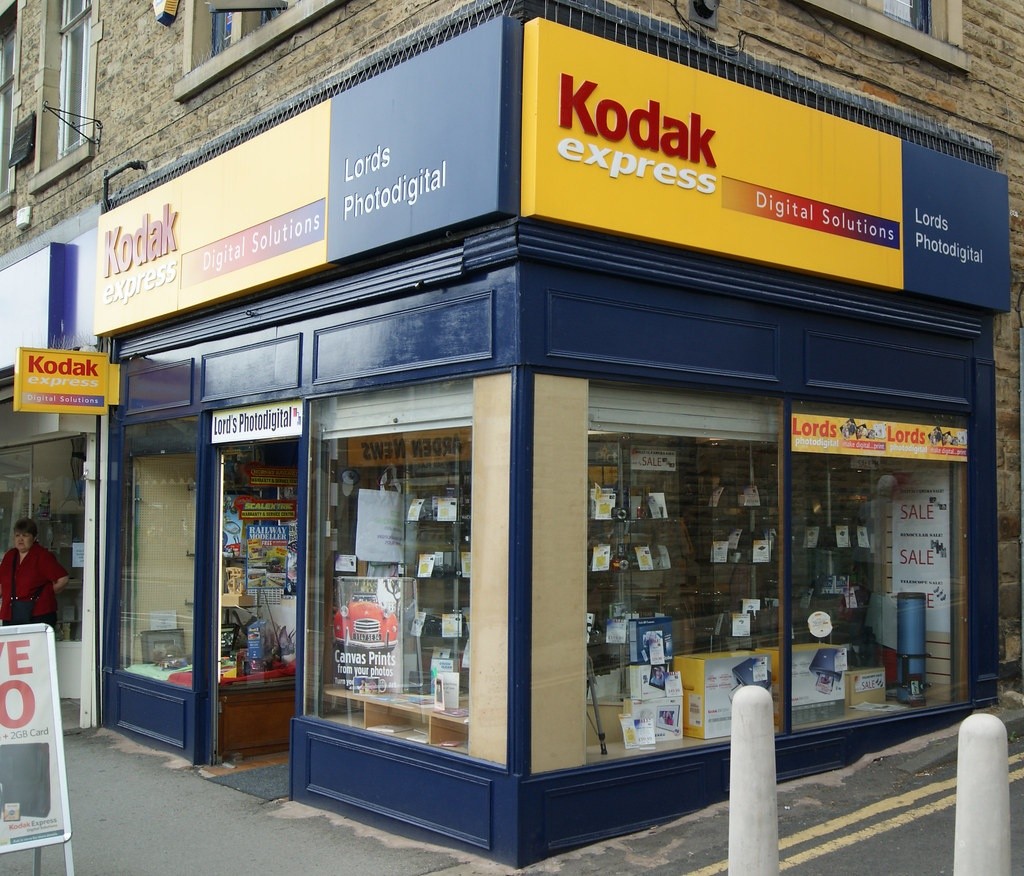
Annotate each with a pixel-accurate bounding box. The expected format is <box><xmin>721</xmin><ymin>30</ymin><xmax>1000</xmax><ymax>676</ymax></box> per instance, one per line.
<box><xmin>0</xmin><ymin>517</ymin><xmax>70</xmax><ymax>628</ymax></box>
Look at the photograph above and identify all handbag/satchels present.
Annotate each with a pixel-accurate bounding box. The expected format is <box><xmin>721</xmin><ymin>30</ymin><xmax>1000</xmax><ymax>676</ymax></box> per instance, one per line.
<box><xmin>10</xmin><ymin>598</ymin><xmax>34</xmax><ymax>625</ymax></box>
<box><xmin>355</xmin><ymin>466</ymin><xmax>417</xmax><ymax>563</ymax></box>
